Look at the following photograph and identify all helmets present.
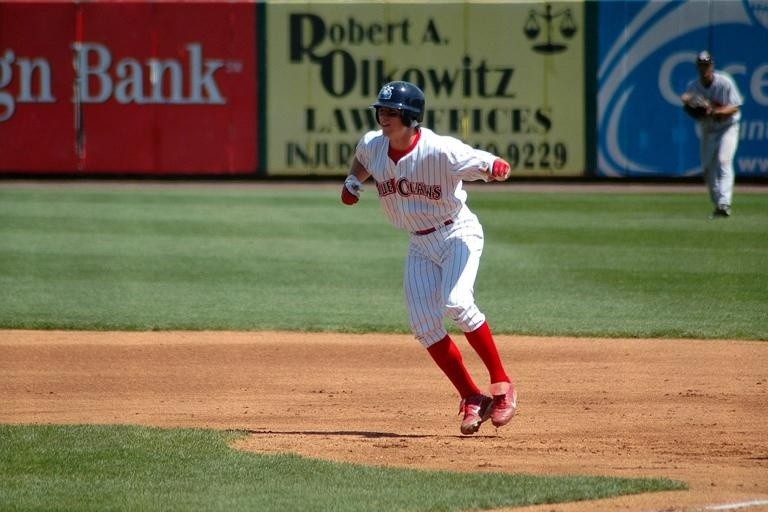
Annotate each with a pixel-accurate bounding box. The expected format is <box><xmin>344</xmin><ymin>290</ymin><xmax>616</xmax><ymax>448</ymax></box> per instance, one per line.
<box><xmin>369</xmin><ymin>81</ymin><xmax>423</xmax><ymax>128</ymax></box>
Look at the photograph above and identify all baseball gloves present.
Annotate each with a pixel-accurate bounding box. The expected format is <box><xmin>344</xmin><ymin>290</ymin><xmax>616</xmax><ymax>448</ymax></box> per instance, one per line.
<box><xmin>683</xmin><ymin>96</ymin><xmax>713</xmax><ymax>118</ymax></box>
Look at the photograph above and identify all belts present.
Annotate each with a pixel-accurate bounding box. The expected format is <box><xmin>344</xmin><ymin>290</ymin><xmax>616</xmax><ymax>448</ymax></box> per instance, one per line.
<box><xmin>415</xmin><ymin>219</ymin><xmax>453</xmax><ymax>237</ymax></box>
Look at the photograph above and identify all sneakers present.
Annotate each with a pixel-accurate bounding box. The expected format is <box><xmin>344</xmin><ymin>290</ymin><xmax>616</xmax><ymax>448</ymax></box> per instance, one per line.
<box><xmin>491</xmin><ymin>382</ymin><xmax>516</xmax><ymax>426</ymax></box>
<box><xmin>461</xmin><ymin>395</ymin><xmax>493</xmax><ymax>433</ymax></box>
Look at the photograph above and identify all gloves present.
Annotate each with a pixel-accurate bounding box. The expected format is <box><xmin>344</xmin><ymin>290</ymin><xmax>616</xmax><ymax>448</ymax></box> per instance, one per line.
<box><xmin>342</xmin><ymin>175</ymin><xmax>365</xmax><ymax>205</ymax></box>
<box><xmin>478</xmin><ymin>158</ymin><xmax>510</xmax><ymax>179</ymax></box>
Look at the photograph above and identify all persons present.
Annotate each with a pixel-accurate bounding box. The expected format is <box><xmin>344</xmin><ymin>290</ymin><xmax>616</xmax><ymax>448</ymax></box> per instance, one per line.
<box><xmin>339</xmin><ymin>79</ymin><xmax>520</xmax><ymax>435</ymax></box>
<box><xmin>681</xmin><ymin>50</ymin><xmax>744</xmax><ymax>216</ymax></box>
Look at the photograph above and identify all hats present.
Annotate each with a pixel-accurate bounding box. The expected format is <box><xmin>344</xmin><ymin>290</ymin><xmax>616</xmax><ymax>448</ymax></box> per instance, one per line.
<box><xmin>696</xmin><ymin>51</ymin><xmax>715</xmax><ymax>64</ymax></box>
